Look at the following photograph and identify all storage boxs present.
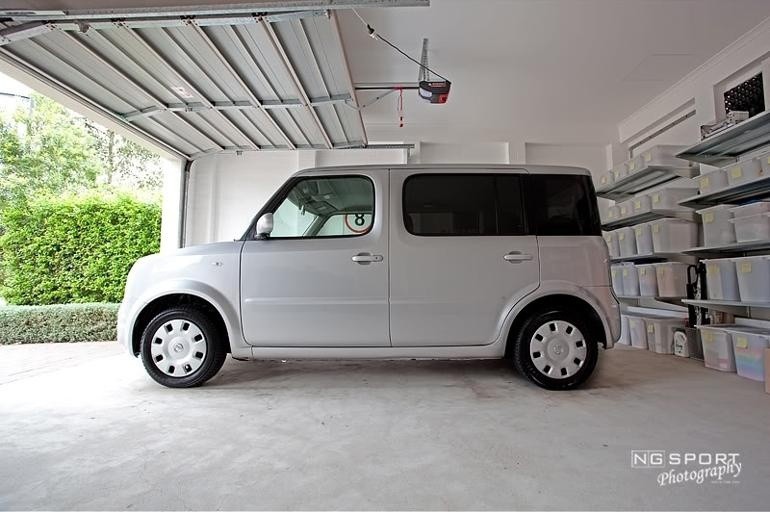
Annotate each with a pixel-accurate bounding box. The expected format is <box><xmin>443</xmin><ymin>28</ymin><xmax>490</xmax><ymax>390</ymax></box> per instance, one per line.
<box><xmin>691</xmin><ymin>151</ymin><xmax>770</xmax><ymax>383</ymax></box>
<box><xmin>592</xmin><ymin>144</ymin><xmax>701</xmax><ymax>355</ymax></box>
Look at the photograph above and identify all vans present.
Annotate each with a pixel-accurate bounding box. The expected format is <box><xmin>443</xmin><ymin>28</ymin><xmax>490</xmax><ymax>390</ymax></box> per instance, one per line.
<box><xmin>116</xmin><ymin>163</ymin><xmax>622</xmax><ymax>391</ymax></box>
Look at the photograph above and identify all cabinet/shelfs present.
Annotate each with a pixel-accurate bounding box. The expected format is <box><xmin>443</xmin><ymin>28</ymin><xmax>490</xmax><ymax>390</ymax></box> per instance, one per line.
<box><xmin>674</xmin><ymin>110</ymin><xmax>770</xmax><ymax>364</ymax></box>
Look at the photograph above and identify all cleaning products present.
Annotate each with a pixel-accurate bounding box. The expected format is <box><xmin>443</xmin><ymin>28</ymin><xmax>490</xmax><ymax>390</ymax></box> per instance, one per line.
<box><xmin>673</xmin><ymin>331</ymin><xmax>689</xmax><ymax>358</ymax></box>
